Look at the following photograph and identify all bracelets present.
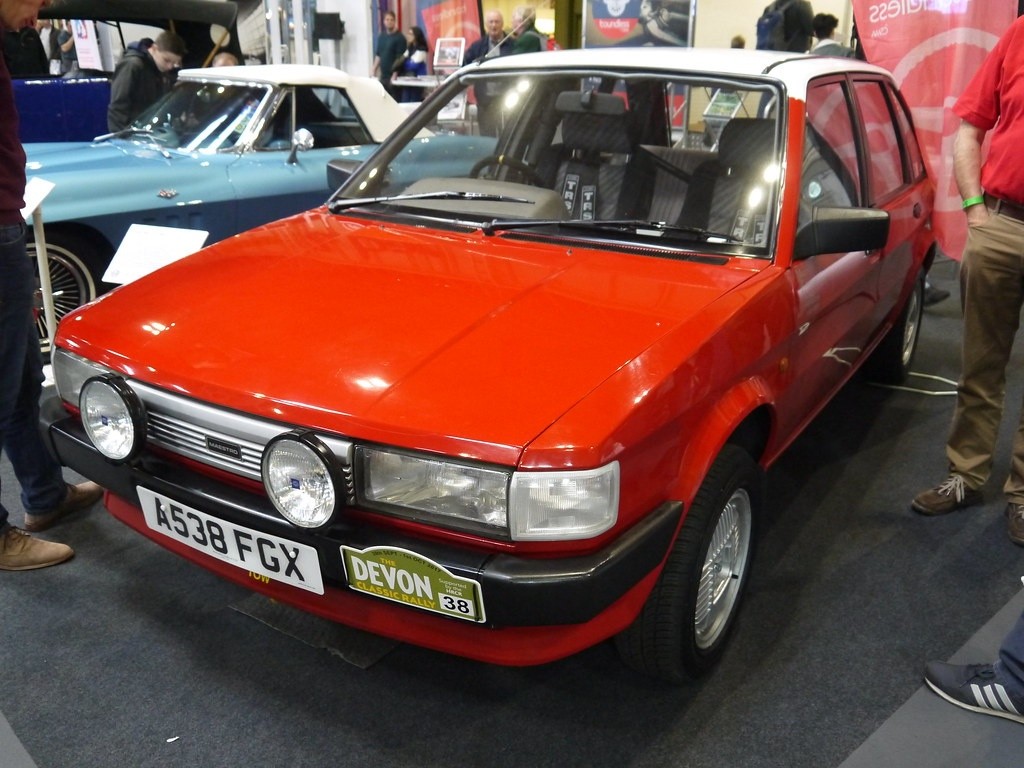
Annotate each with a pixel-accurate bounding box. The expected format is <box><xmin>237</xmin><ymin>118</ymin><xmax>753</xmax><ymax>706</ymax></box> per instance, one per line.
<box><xmin>962</xmin><ymin>195</ymin><xmax>984</xmax><ymax>212</ymax></box>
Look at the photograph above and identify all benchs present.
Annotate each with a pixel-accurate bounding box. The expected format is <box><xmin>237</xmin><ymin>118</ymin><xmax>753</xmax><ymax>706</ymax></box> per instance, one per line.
<box><xmin>637</xmin><ymin>143</ymin><xmax>719</xmax><ymax>225</ymax></box>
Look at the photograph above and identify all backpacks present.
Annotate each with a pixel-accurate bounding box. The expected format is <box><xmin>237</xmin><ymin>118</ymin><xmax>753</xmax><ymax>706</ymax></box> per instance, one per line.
<box><xmin>522</xmin><ymin>30</ymin><xmax>564</xmax><ymax>51</ymax></box>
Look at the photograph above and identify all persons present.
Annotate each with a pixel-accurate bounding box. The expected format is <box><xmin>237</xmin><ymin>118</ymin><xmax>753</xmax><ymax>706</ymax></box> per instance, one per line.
<box><xmin>38</xmin><ymin>11</ymin><xmax>542</xmax><ymax>133</ymax></box>
<box><xmin>911</xmin><ymin>15</ymin><xmax>1024</xmax><ymax>544</ymax></box>
<box><xmin>0</xmin><ymin>1</ymin><xmax>102</xmax><ymax>572</ymax></box>
<box><xmin>923</xmin><ymin>590</ymin><xmax>1024</xmax><ymax>725</ymax></box>
<box><xmin>806</xmin><ymin>13</ymin><xmax>858</xmax><ymax>58</ymax></box>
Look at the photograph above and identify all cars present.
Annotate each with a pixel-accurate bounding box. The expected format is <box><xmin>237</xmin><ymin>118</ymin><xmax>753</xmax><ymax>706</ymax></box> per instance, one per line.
<box><xmin>19</xmin><ymin>62</ymin><xmax>505</xmax><ymax>370</ymax></box>
<box><xmin>42</xmin><ymin>46</ymin><xmax>938</xmax><ymax>688</ymax></box>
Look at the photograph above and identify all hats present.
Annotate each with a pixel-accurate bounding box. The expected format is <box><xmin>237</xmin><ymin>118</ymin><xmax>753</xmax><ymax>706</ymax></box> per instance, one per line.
<box><xmin>513</xmin><ymin>4</ymin><xmax>537</xmax><ymax>24</ymax></box>
<box><xmin>58</xmin><ymin>30</ymin><xmax>71</xmax><ymax>46</ymax></box>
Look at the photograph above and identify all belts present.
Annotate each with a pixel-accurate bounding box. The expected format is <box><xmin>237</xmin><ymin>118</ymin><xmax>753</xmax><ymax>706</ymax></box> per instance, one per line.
<box><xmin>982</xmin><ymin>191</ymin><xmax>1024</xmax><ymax>221</ymax></box>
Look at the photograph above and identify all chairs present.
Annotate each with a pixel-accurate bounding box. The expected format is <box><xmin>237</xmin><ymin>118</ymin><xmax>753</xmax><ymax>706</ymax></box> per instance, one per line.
<box><xmin>538</xmin><ymin>89</ymin><xmax>634</xmax><ymax>226</ymax></box>
<box><xmin>697</xmin><ymin>116</ymin><xmax>780</xmax><ymax>248</ymax></box>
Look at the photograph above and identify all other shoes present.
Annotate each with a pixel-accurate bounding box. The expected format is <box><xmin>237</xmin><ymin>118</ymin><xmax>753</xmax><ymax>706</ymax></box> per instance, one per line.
<box><xmin>923</xmin><ymin>285</ymin><xmax>950</xmax><ymax>304</ymax></box>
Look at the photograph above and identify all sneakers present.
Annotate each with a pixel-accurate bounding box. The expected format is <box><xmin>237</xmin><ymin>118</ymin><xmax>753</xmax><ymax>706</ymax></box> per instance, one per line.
<box><xmin>0</xmin><ymin>521</ymin><xmax>73</xmax><ymax>570</ymax></box>
<box><xmin>1003</xmin><ymin>502</ymin><xmax>1024</xmax><ymax>543</ymax></box>
<box><xmin>923</xmin><ymin>660</ymin><xmax>1024</xmax><ymax>724</ymax></box>
<box><xmin>25</xmin><ymin>480</ymin><xmax>105</xmax><ymax>531</ymax></box>
<box><xmin>911</xmin><ymin>472</ymin><xmax>983</xmax><ymax>515</ymax></box>
